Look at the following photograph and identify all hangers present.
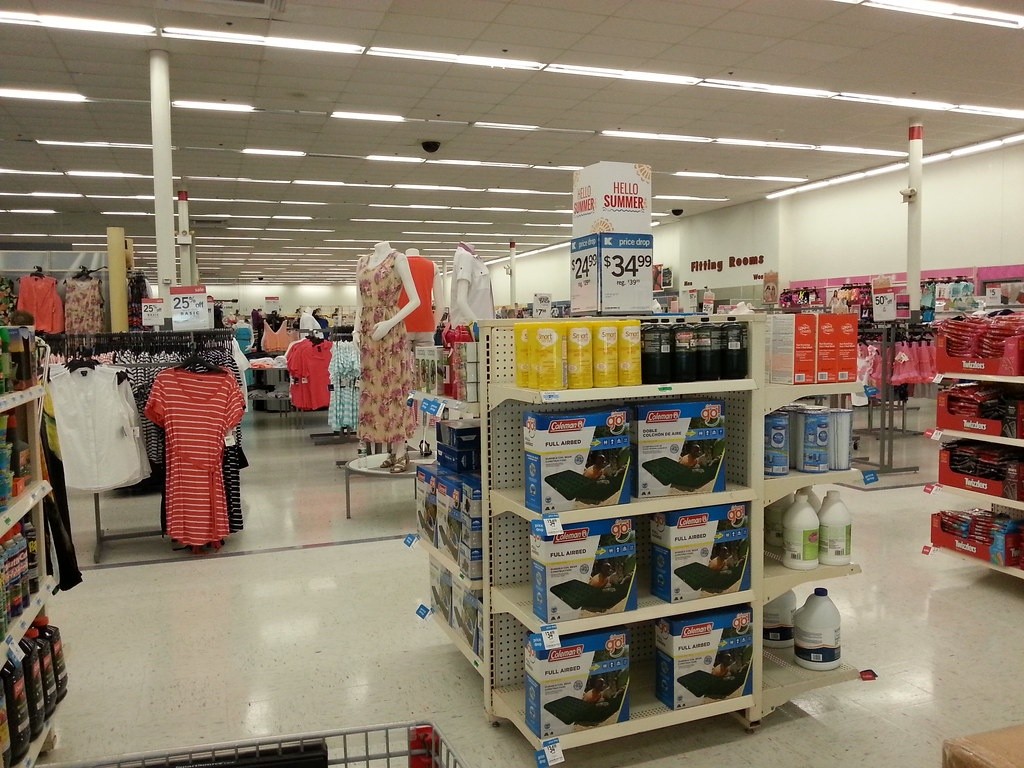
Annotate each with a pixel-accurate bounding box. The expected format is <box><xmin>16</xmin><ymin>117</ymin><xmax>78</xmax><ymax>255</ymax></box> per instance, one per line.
<box><xmin>15</xmin><ymin>265</ymin><xmax>146</xmax><ymax>285</ymax></box>
<box><xmin>840</xmin><ymin>282</ymin><xmax>871</xmax><ymax>290</ymax></box>
<box><xmin>331</xmin><ymin>308</ymin><xmax>338</xmax><ymax>319</ymax></box>
<box><xmin>305</xmin><ymin>325</ymin><xmax>353</xmax><ymax>347</ymax></box>
<box><xmin>857</xmin><ymin>323</ymin><xmax>934</xmax><ymax>355</ymax></box>
<box><xmin>266</xmin><ymin>310</ymin><xmax>285</xmax><ymax>324</ymax></box>
<box><xmin>780</xmin><ymin>285</ymin><xmax>820</xmax><ymax>299</ymax></box>
<box><xmin>920</xmin><ymin>275</ymin><xmax>968</xmax><ymax>288</ymax></box>
<box><xmin>50</xmin><ymin>328</ymin><xmax>234</xmax><ymax>386</ymax></box>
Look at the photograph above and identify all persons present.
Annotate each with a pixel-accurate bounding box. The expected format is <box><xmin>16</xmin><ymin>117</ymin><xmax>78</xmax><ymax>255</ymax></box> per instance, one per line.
<box><xmin>232</xmin><ymin>319</ymin><xmax>255</xmax><ymax>387</ymax></box>
<box><xmin>449</xmin><ymin>241</ymin><xmax>496</xmax><ymax>329</ymax></box>
<box><xmin>397</xmin><ymin>247</ymin><xmax>446</xmax><ymax>360</ymax></box>
<box><xmin>222</xmin><ymin>312</ymin><xmax>237</xmax><ymax>329</ymax></box>
<box><xmin>352</xmin><ymin>240</ymin><xmax>421</xmax><ymax>473</ymax></box>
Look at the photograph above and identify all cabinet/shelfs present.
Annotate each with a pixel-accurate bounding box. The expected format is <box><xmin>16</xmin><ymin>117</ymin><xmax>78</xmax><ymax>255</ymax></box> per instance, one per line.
<box><xmin>245</xmin><ymin>368</ymin><xmax>291</xmax><ymax>401</ymax></box>
<box><xmin>409</xmin><ymin>307</ymin><xmax>862</xmax><ymax>768</ymax></box>
<box><xmin>0</xmin><ymin>384</ymin><xmax>58</xmax><ymax>768</ymax></box>
<box><xmin>930</xmin><ymin>372</ymin><xmax>1024</xmax><ymax>580</ymax></box>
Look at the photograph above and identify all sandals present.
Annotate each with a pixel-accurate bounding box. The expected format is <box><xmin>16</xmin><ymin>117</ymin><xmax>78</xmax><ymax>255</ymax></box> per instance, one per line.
<box><xmin>390</xmin><ymin>453</ymin><xmax>409</xmax><ymax>472</ymax></box>
<box><xmin>380</xmin><ymin>453</ymin><xmax>396</xmax><ymax>468</ymax></box>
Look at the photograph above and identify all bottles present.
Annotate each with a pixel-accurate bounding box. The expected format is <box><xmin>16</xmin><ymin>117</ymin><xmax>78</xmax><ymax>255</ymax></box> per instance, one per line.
<box><xmin>764</xmin><ymin>491</ymin><xmax>794</xmax><ymax>547</ymax></box>
<box><xmin>0</xmin><ymin>520</ymin><xmax>40</xmax><ymax>644</ymax></box>
<box><xmin>782</xmin><ymin>494</ymin><xmax>819</xmax><ymax>570</ymax></box>
<box><xmin>641</xmin><ymin>282</ymin><xmax>752</xmax><ymax>384</ymax></box>
<box><xmin>793</xmin><ymin>588</ymin><xmax>841</xmax><ymax>671</ymax></box>
<box><xmin>32</xmin><ymin>615</ymin><xmax>68</xmax><ymax>694</ymax></box>
<box><xmin>763</xmin><ymin>589</ymin><xmax>796</xmax><ymax>648</ymax></box>
<box><xmin>0</xmin><ymin>656</ymin><xmax>30</xmax><ymax>768</ymax></box>
<box><xmin>797</xmin><ymin>485</ymin><xmax>822</xmax><ymax>515</ymax></box>
<box><xmin>19</xmin><ymin>637</ymin><xmax>44</xmax><ymax>742</ymax></box>
<box><xmin>26</xmin><ymin>628</ymin><xmax>57</xmax><ymax>713</ymax></box>
<box><xmin>818</xmin><ymin>490</ymin><xmax>852</xmax><ymax>565</ymax></box>
<box><xmin>7</xmin><ymin>409</ymin><xmax>30</xmax><ymax>485</ymax></box>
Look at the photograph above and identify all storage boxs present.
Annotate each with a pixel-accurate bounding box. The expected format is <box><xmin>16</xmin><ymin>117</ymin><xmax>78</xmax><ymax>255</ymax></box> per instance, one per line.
<box><xmin>935</xmin><ymin>335</ymin><xmax>1024</xmax><ymax>376</ymax></box>
<box><xmin>0</xmin><ymin>325</ymin><xmax>36</xmax><ymax>390</ymax></box>
<box><xmin>622</xmin><ymin>396</ymin><xmax>727</xmax><ymax>499</ymax></box>
<box><xmin>524</xmin><ymin>624</ymin><xmax>631</xmax><ymax>740</ymax></box>
<box><xmin>529</xmin><ymin>516</ymin><xmax>638</xmax><ymax>624</ymax></box>
<box><xmin>936</xmin><ymin>391</ymin><xmax>1024</xmax><ymax>439</ymax></box>
<box><xmin>648</xmin><ymin>503</ymin><xmax>751</xmax><ymax>604</ymax></box>
<box><xmin>939</xmin><ymin>449</ymin><xmax>1024</xmax><ymax>501</ymax></box>
<box><xmin>522</xmin><ymin>404</ymin><xmax>631</xmax><ymax>515</ymax></box>
<box><xmin>931</xmin><ymin>513</ymin><xmax>1020</xmax><ymax>567</ymax></box>
<box><xmin>765</xmin><ymin>314</ymin><xmax>858</xmax><ymax>386</ymax></box>
<box><xmin>654</xmin><ymin>602</ymin><xmax>752</xmax><ymax>711</ymax></box>
<box><xmin>415</xmin><ymin>342</ymin><xmax>484</xmax><ymax>662</ymax></box>
